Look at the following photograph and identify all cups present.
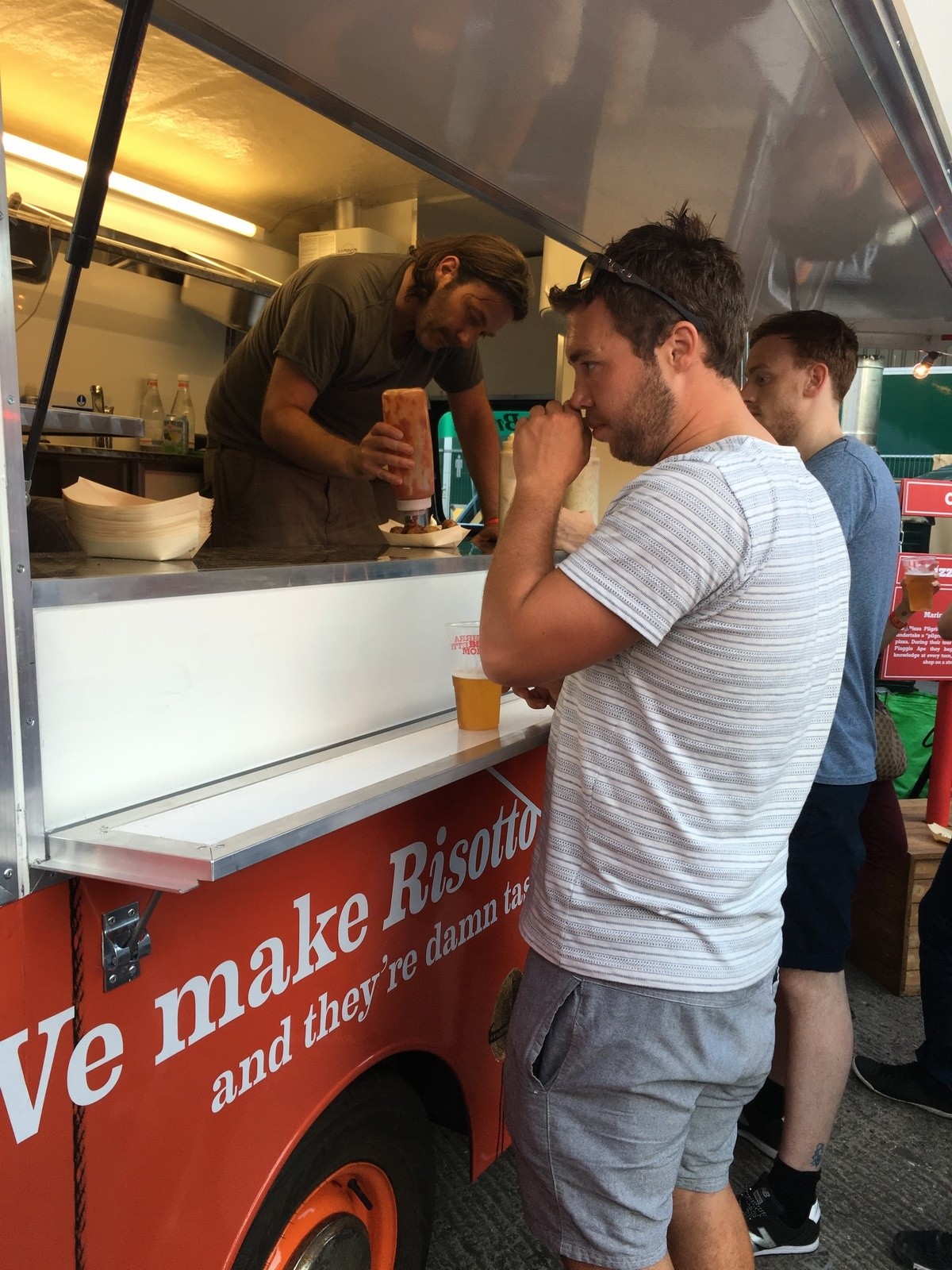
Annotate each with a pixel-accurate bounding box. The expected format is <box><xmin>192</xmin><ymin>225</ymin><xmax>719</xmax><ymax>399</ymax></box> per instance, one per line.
<box><xmin>448</xmin><ymin>620</ymin><xmax>503</xmax><ymax>731</ymax></box>
<box><xmin>901</xmin><ymin>556</ymin><xmax>936</xmax><ymax>612</ymax></box>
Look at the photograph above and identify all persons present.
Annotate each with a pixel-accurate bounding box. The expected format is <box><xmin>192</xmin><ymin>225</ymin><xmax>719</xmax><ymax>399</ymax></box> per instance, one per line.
<box><xmin>852</xmin><ymin>561</ymin><xmax>952</xmax><ymax>1270</ymax></box>
<box><xmin>736</xmin><ymin>310</ymin><xmax>900</xmax><ymax>1256</ymax></box>
<box><xmin>204</xmin><ymin>234</ymin><xmax>532</xmax><ymax>560</ymax></box>
<box><xmin>478</xmin><ymin>198</ymin><xmax>852</xmax><ymax>1270</ymax></box>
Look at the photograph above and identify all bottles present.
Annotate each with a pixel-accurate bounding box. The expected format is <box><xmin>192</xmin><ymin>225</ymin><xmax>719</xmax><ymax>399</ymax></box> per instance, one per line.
<box><xmin>498</xmin><ymin>432</ymin><xmax>515</xmax><ymax>540</ymax></box>
<box><xmin>563</xmin><ymin>443</ymin><xmax>601</xmax><ymax>528</ymax></box>
<box><xmin>139</xmin><ymin>372</ymin><xmax>166</xmax><ymax>453</ymax></box>
<box><xmin>382</xmin><ymin>387</ymin><xmax>436</xmax><ymax>527</ymax></box>
<box><xmin>170</xmin><ymin>375</ymin><xmax>196</xmax><ymax>453</ymax></box>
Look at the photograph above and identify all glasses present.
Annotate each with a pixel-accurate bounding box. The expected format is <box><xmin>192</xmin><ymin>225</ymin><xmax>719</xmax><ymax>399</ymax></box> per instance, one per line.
<box><xmin>575</xmin><ymin>252</ymin><xmax>705</xmax><ymax>335</ymax></box>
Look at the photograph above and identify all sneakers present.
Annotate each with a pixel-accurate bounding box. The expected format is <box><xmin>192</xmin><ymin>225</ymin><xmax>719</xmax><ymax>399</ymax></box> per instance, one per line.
<box><xmin>892</xmin><ymin>1229</ymin><xmax>952</xmax><ymax>1270</ymax></box>
<box><xmin>738</xmin><ymin>1077</ymin><xmax>788</xmax><ymax>1160</ymax></box>
<box><xmin>734</xmin><ymin>1171</ymin><xmax>821</xmax><ymax>1256</ymax></box>
<box><xmin>852</xmin><ymin>1054</ymin><xmax>952</xmax><ymax>1118</ymax></box>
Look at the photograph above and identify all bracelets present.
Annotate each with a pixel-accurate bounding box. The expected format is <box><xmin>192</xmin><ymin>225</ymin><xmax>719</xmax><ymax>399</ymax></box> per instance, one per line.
<box><xmin>890</xmin><ymin>611</ymin><xmax>907</xmax><ymax>630</ymax></box>
<box><xmin>485</xmin><ymin>517</ymin><xmax>499</xmax><ymax>527</ymax></box>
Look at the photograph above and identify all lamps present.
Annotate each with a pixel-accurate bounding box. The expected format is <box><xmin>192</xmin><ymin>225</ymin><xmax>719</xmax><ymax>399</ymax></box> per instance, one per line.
<box><xmin>3</xmin><ymin>132</ymin><xmax>267</xmax><ymax>242</ymax></box>
